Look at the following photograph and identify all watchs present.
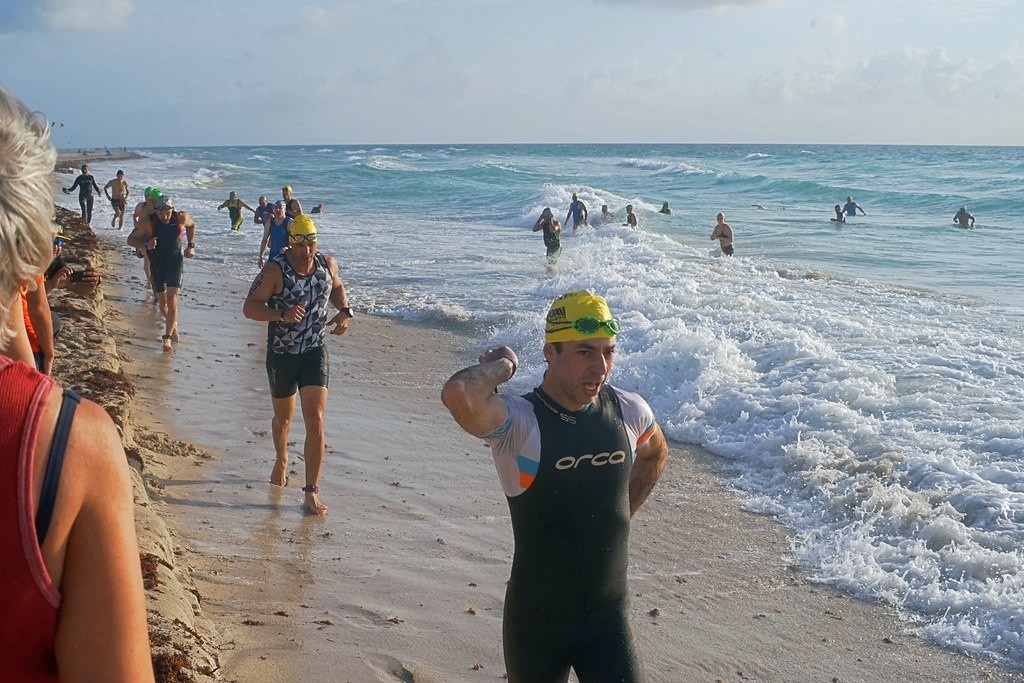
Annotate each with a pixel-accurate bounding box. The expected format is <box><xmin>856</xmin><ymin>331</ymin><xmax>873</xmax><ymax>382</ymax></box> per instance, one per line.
<box><xmin>188</xmin><ymin>243</ymin><xmax>195</xmax><ymax>248</ymax></box>
<box><xmin>342</xmin><ymin>307</ymin><xmax>354</xmax><ymax>317</ymax></box>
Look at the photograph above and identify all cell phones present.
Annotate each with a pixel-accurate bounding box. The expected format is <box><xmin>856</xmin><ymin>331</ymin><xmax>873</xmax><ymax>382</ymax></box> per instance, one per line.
<box><xmin>70</xmin><ymin>270</ymin><xmax>92</xmax><ymax>282</ymax></box>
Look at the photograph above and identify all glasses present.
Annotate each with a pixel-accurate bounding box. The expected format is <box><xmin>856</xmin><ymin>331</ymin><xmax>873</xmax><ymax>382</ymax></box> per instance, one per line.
<box><xmin>571</xmin><ymin>316</ymin><xmax>622</xmax><ymax>336</ymax></box>
<box><xmin>159</xmin><ymin>206</ymin><xmax>172</xmax><ymax>211</ymax></box>
<box><xmin>52</xmin><ymin>237</ymin><xmax>63</xmax><ymax>246</ymax></box>
<box><xmin>293</xmin><ymin>233</ymin><xmax>317</xmax><ymax>243</ymax></box>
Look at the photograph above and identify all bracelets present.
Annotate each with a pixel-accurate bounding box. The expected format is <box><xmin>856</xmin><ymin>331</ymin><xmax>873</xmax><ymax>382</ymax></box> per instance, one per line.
<box><xmin>504</xmin><ymin>356</ymin><xmax>517</xmax><ymax>381</ymax></box>
<box><xmin>280</xmin><ymin>309</ymin><xmax>287</xmax><ymax>323</ymax></box>
<box><xmin>91</xmin><ymin>277</ymin><xmax>101</xmax><ymax>287</ymax></box>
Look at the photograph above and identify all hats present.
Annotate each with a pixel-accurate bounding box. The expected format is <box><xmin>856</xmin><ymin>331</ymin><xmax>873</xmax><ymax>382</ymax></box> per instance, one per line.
<box><xmin>572</xmin><ymin>192</ymin><xmax>579</xmax><ymax>197</ymax></box>
<box><xmin>157</xmin><ymin>195</ymin><xmax>172</xmax><ymax>207</ymax></box>
<box><xmin>283</xmin><ymin>186</ymin><xmax>292</xmax><ymax>194</ymax></box>
<box><xmin>275</xmin><ymin>200</ymin><xmax>286</xmax><ymax>209</ymax></box>
<box><xmin>289</xmin><ymin>215</ymin><xmax>317</xmax><ymax>243</ymax></box>
<box><xmin>144</xmin><ymin>186</ymin><xmax>154</xmax><ymax>194</ymax></box>
<box><xmin>53</xmin><ymin>225</ymin><xmax>71</xmax><ymax>241</ymax></box>
<box><xmin>149</xmin><ymin>187</ymin><xmax>162</xmax><ymax>200</ymax></box>
<box><xmin>626</xmin><ymin>204</ymin><xmax>633</xmax><ymax>208</ymax></box>
<box><xmin>545</xmin><ymin>289</ymin><xmax>618</xmax><ymax>344</ymax></box>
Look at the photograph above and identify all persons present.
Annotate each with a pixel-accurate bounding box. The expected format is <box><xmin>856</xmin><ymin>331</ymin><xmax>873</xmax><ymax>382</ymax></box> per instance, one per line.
<box><xmin>953</xmin><ymin>206</ymin><xmax>976</xmax><ymax>226</ymax></box>
<box><xmin>533</xmin><ymin>208</ymin><xmax>562</xmax><ymax>266</ymax></box>
<box><xmin>218</xmin><ymin>186</ymin><xmax>302</xmax><ymax>268</ymax></box>
<box><xmin>243</xmin><ymin>214</ymin><xmax>354</xmax><ymax>514</ymax></box>
<box><xmin>565</xmin><ymin>192</ymin><xmax>637</xmax><ymax>230</ymax></box>
<box><xmin>62</xmin><ymin>165</ymin><xmax>102</xmax><ymax>224</ymax></box>
<box><xmin>831</xmin><ymin>197</ymin><xmax>866</xmax><ymax>223</ymax></box>
<box><xmin>0</xmin><ymin>89</ymin><xmax>156</xmax><ymax>683</ymax></box>
<box><xmin>104</xmin><ymin>170</ymin><xmax>130</xmax><ymax>229</ymax></box>
<box><xmin>659</xmin><ymin>202</ymin><xmax>671</xmax><ymax>214</ymax></box>
<box><xmin>711</xmin><ymin>214</ymin><xmax>734</xmax><ymax>257</ymax></box>
<box><xmin>6</xmin><ymin>225</ymin><xmax>102</xmax><ymax>378</ymax></box>
<box><xmin>127</xmin><ymin>187</ymin><xmax>196</xmax><ymax>349</ymax></box>
<box><xmin>441</xmin><ymin>291</ymin><xmax>669</xmax><ymax>683</ymax></box>
<box><xmin>312</xmin><ymin>204</ymin><xmax>323</xmax><ymax>214</ymax></box>
